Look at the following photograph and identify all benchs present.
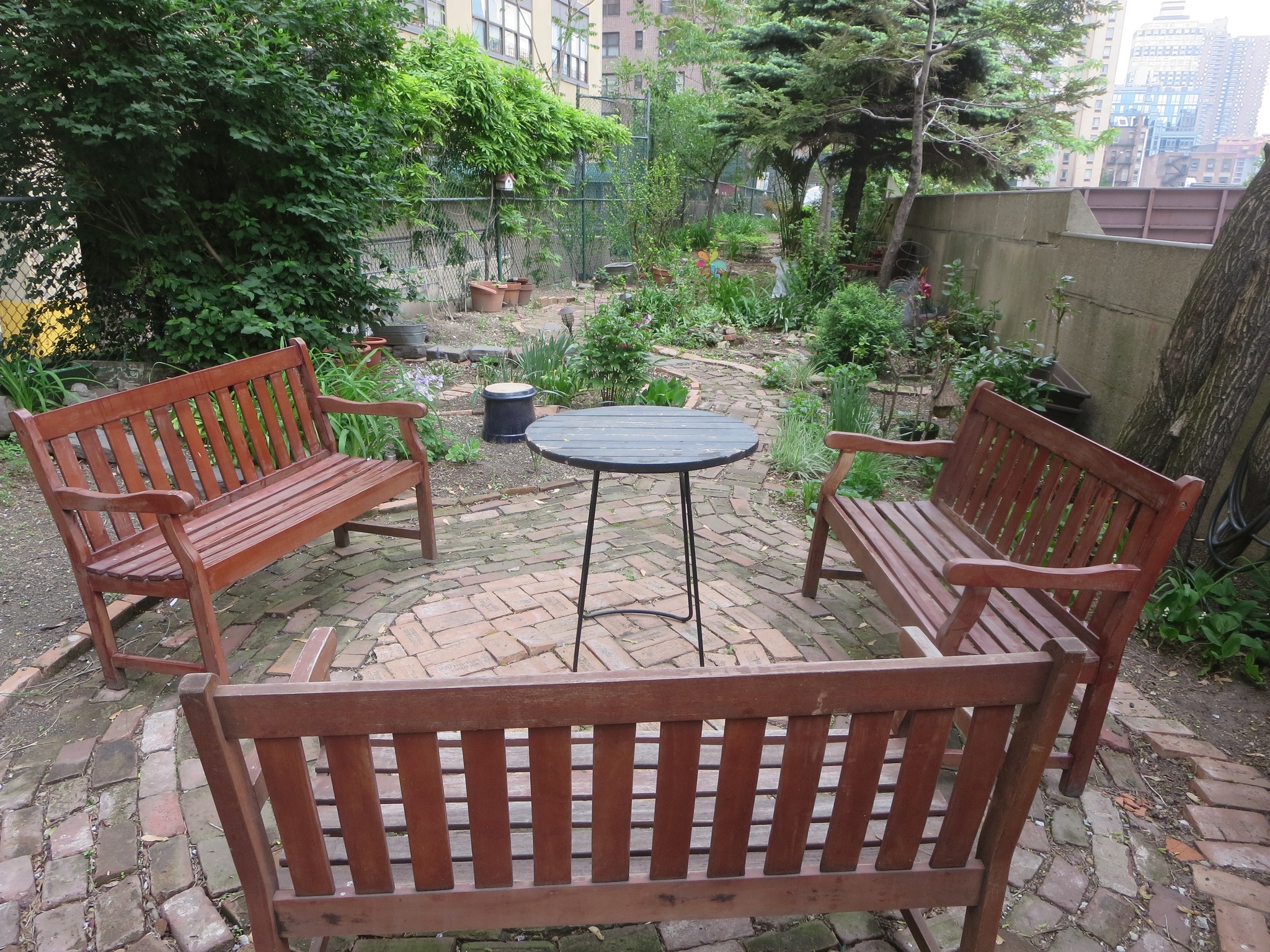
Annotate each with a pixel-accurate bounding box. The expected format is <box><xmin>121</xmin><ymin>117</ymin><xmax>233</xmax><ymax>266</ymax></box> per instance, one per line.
<box><xmin>802</xmin><ymin>381</ymin><xmax>1205</xmax><ymax>798</ymax></box>
<box><xmin>8</xmin><ymin>338</ymin><xmax>438</xmax><ymax>690</ymax></box>
<box><xmin>177</xmin><ymin>622</ymin><xmax>1089</xmax><ymax>952</ymax></box>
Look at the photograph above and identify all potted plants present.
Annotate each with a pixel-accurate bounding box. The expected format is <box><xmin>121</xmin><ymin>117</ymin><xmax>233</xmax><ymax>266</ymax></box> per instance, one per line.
<box><xmin>653</xmin><ymin>245</ymin><xmax>682</xmax><ymax>288</ymax></box>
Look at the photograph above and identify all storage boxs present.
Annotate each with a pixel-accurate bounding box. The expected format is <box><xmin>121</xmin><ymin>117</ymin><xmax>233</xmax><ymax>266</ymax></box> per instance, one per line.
<box><xmin>995</xmin><ymin>345</ymin><xmax>1092</xmax><ymax>428</ymax></box>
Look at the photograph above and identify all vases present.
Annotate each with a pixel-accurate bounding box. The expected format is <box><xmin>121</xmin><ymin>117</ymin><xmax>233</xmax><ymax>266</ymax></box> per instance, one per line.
<box><xmin>322</xmin><ymin>337</ymin><xmax>387</xmax><ymax>396</ymax></box>
<box><xmin>467</xmin><ymin>277</ymin><xmax>533</xmax><ymax>313</ymax></box>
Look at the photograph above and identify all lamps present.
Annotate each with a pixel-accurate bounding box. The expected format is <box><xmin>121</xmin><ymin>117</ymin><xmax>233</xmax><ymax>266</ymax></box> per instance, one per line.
<box><xmin>557</xmin><ymin>307</ymin><xmax>577</xmax><ymax>344</ymax></box>
<box><xmin>617</xmin><ymin>293</ymin><xmax>633</xmax><ymax>314</ymax></box>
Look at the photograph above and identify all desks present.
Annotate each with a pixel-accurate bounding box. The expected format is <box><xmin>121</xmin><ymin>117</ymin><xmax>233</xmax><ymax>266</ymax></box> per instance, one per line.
<box><xmin>524</xmin><ymin>406</ymin><xmax>760</xmax><ymax>673</ymax></box>
